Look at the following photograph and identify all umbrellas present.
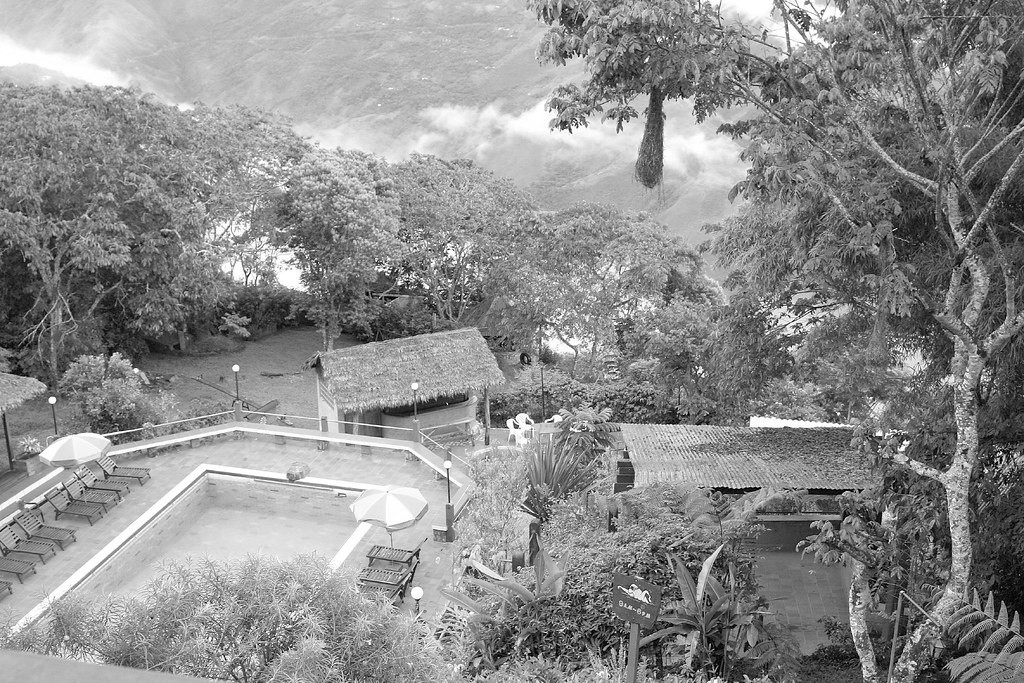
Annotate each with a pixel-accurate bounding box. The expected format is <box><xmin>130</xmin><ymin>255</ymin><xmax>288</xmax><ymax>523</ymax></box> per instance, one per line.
<box><xmin>39</xmin><ymin>433</ymin><xmax>111</xmax><ymax>503</ymax></box>
<box><xmin>349</xmin><ymin>484</ymin><xmax>429</xmax><ymax>567</ymax></box>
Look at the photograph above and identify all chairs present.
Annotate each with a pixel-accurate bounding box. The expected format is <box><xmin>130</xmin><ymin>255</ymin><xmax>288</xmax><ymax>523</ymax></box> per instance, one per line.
<box><xmin>506</xmin><ymin>418</ymin><xmax>526</xmax><ymax>446</ymax></box>
<box><xmin>356</xmin><ymin>584</ymin><xmax>405</xmax><ymax>610</ymax></box>
<box><xmin>44</xmin><ymin>487</ymin><xmax>105</xmax><ymax>526</ymax></box>
<box><xmin>63</xmin><ymin>475</ymin><xmax>118</xmax><ymax>513</ymax></box>
<box><xmin>0</xmin><ymin>556</ymin><xmax>37</xmax><ymax>584</ymax></box>
<box><xmin>367</xmin><ymin>537</ymin><xmax>428</xmax><ymax>569</ymax></box>
<box><xmin>515</xmin><ymin>413</ymin><xmax>534</xmax><ymax>440</ymax></box>
<box><xmin>355</xmin><ymin>560</ymin><xmax>421</xmax><ymax>598</ymax></box>
<box><xmin>95</xmin><ymin>455</ymin><xmax>151</xmax><ymax>485</ymax></box>
<box><xmin>545</xmin><ymin>414</ymin><xmax>563</xmax><ymax>423</ymax></box>
<box><xmin>0</xmin><ymin>522</ymin><xmax>56</xmax><ymax>565</ymax></box>
<box><xmin>0</xmin><ymin>580</ymin><xmax>13</xmax><ymax>595</ymax></box>
<box><xmin>73</xmin><ymin>464</ymin><xmax>130</xmax><ymax>500</ymax></box>
<box><xmin>13</xmin><ymin>508</ymin><xmax>77</xmax><ymax>551</ymax></box>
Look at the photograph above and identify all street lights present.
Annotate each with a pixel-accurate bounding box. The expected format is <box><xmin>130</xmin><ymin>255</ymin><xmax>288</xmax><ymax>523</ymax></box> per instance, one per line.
<box><xmin>411</xmin><ymin>382</ymin><xmax>421</xmax><ymax>441</ymax></box>
<box><xmin>48</xmin><ymin>397</ymin><xmax>61</xmax><ymax>441</ymax></box>
<box><xmin>232</xmin><ymin>364</ymin><xmax>242</xmax><ymax>422</ymax></box>
<box><xmin>444</xmin><ymin>460</ymin><xmax>456</xmax><ymax>542</ymax></box>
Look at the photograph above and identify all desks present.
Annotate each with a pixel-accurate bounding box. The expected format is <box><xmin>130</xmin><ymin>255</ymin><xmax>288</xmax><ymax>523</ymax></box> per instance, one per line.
<box><xmin>533</xmin><ymin>423</ymin><xmax>563</xmax><ymax>448</ymax></box>
